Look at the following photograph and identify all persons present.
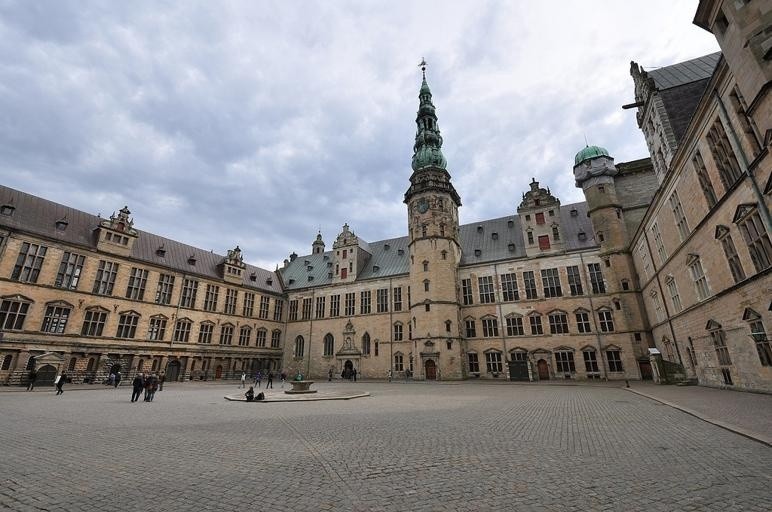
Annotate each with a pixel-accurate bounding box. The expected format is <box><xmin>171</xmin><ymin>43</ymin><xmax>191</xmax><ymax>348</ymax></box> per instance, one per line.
<box><xmin>240</xmin><ymin>371</ymin><xmax>247</xmax><ymax>387</ymax></box>
<box><xmin>254</xmin><ymin>371</ymin><xmax>261</xmax><ymax>389</ymax></box>
<box><xmin>27</xmin><ymin>369</ymin><xmax>39</xmax><ymax>391</ymax></box>
<box><xmin>386</xmin><ymin>370</ymin><xmax>391</xmax><ymax>382</ymax></box>
<box><xmin>341</xmin><ymin>366</ymin><xmax>357</xmax><ymax>382</ymax></box>
<box><xmin>405</xmin><ymin>368</ymin><xmax>410</xmax><ymax>380</ymax></box>
<box><xmin>56</xmin><ymin>370</ymin><xmax>68</xmax><ymax>395</ymax></box>
<box><xmin>328</xmin><ymin>368</ymin><xmax>332</xmax><ymax>381</ymax></box>
<box><xmin>280</xmin><ymin>372</ymin><xmax>288</xmax><ymax>388</ymax></box>
<box><xmin>265</xmin><ymin>371</ymin><xmax>273</xmax><ymax>389</ymax></box>
<box><xmin>244</xmin><ymin>386</ymin><xmax>254</xmax><ymax>402</ymax></box>
<box><xmin>109</xmin><ymin>369</ymin><xmax>167</xmax><ymax>402</ymax></box>
